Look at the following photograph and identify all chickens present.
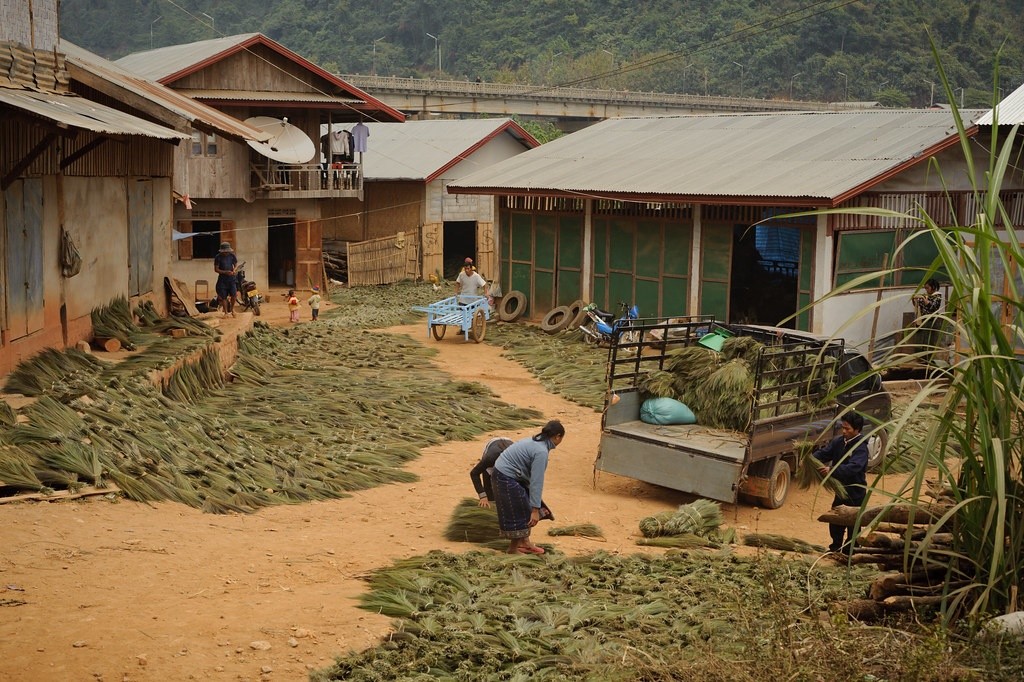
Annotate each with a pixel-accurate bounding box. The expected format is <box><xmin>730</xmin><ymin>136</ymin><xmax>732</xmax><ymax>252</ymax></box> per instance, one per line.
<box><xmin>330</xmin><ymin>278</ymin><xmax>343</xmax><ymax>286</ymax></box>
<box><xmin>433</xmin><ymin>284</ymin><xmax>442</xmax><ymax>291</ymax></box>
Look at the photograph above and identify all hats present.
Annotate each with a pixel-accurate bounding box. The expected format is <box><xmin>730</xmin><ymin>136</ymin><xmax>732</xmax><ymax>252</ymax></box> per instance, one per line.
<box><xmin>465</xmin><ymin>258</ymin><xmax>473</xmax><ymax>263</ymax></box>
<box><xmin>311</xmin><ymin>285</ymin><xmax>320</xmax><ymax>294</ymax></box>
<box><xmin>219</xmin><ymin>242</ymin><xmax>235</xmax><ymax>252</ymax></box>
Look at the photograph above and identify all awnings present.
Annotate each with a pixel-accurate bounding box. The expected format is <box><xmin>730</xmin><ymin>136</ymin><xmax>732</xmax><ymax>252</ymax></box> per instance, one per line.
<box><xmin>57</xmin><ymin>35</ymin><xmax>276</xmax><ymax>178</ymax></box>
<box><xmin>0</xmin><ymin>89</ymin><xmax>199</xmax><ymax>303</ymax></box>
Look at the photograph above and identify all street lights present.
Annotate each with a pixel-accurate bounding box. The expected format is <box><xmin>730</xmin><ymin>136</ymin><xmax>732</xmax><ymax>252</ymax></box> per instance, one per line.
<box><xmin>878</xmin><ymin>80</ymin><xmax>890</xmax><ymax>91</ymax></box>
<box><xmin>150</xmin><ymin>14</ymin><xmax>163</xmax><ymax>49</ymax></box>
<box><xmin>604</xmin><ymin>49</ymin><xmax>613</xmax><ymax>89</ymax></box>
<box><xmin>551</xmin><ymin>51</ymin><xmax>564</xmax><ymax>65</ymax></box>
<box><xmin>953</xmin><ymin>86</ymin><xmax>962</xmax><ymax>97</ymax></box>
<box><xmin>923</xmin><ymin>79</ymin><xmax>934</xmax><ymax>107</ymax></box>
<box><xmin>683</xmin><ymin>63</ymin><xmax>694</xmax><ymax>93</ymax></box>
<box><xmin>790</xmin><ymin>72</ymin><xmax>805</xmax><ymax>100</ymax></box>
<box><xmin>733</xmin><ymin>61</ymin><xmax>743</xmax><ymax>96</ymax></box>
<box><xmin>203</xmin><ymin>12</ymin><xmax>215</xmax><ymax>38</ymax></box>
<box><xmin>372</xmin><ymin>36</ymin><xmax>387</xmax><ymax>75</ymax></box>
<box><xmin>997</xmin><ymin>86</ymin><xmax>1003</xmax><ymax>100</ymax></box>
<box><xmin>838</xmin><ymin>71</ymin><xmax>847</xmax><ymax>99</ymax></box>
<box><xmin>426</xmin><ymin>32</ymin><xmax>437</xmax><ymax>78</ymax></box>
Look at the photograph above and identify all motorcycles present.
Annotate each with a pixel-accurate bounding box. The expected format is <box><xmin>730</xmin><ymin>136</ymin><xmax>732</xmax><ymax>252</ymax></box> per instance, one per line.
<box><xmin>233</xmin><ymin>260</ymin><xmax>263</xmax><ymax>317</ymax></box>
<box><xmin>578</xmin><ymin>297</ymin><xmax>646</xmax><ymax>352</ymax></box>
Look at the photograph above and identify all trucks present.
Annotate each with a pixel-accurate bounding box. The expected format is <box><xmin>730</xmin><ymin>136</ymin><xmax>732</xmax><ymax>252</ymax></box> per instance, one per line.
<box><xmin>592</xmin><ymin>313</ymin><xmax>893</xmax><ymax>511</ymax></box>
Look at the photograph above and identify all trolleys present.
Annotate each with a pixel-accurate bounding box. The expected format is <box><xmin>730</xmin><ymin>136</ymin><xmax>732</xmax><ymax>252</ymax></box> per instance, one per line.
<box><xmin>410</xmin><ymin>294</ymin><xmax>490</xmax><ymax>344</ymax></box>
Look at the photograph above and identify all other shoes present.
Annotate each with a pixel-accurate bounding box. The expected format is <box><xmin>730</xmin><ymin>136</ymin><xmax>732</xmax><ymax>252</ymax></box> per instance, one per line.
<box><xmin>511</xmin><ymin>542</ymin><xmax>544</xmax><ymax>554</ymax></box>
<box><xmin>457</xmin><ymin>329</ymin><xmax>464</xmax><ymax>334</ymax></box>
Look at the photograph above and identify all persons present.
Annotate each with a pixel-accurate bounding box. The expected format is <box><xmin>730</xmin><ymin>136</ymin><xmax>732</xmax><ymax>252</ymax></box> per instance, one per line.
<box><xmin>461</xmin><ymin>73</ymin><xmax>482</xmax><ymax>82</ymax></box>
<box><xmin>910</xmin><ymin>275</ymin><xmax>943</xmax><ymax>374</ymax></box>
<box><xmin>214</xmin><ymin>241</ymin><xmax>238</xmax><ymax>319</ymax></box>
<box><xmin>307</xmin><ymin>287</ymin><xmax>321</xmax><ymax>321</ymax></box>
<box><xmin>452</xmin><ymin>258</ymin><xmax>491</xmax><ymax>335</ymax></box>
<box><xmin>797</xmin><ymin>412</ymin><xmax>871</xmax><ymax>556</ymax></box>
<box><xmin>470</xmin><ymin>421</ymin><xmax>565</xmax><ymax>556</ymax></box>
<box><xmin>287</xmin><ymin>289</ymin><xmax>301</xmax><ymax>323</ymax></box>
